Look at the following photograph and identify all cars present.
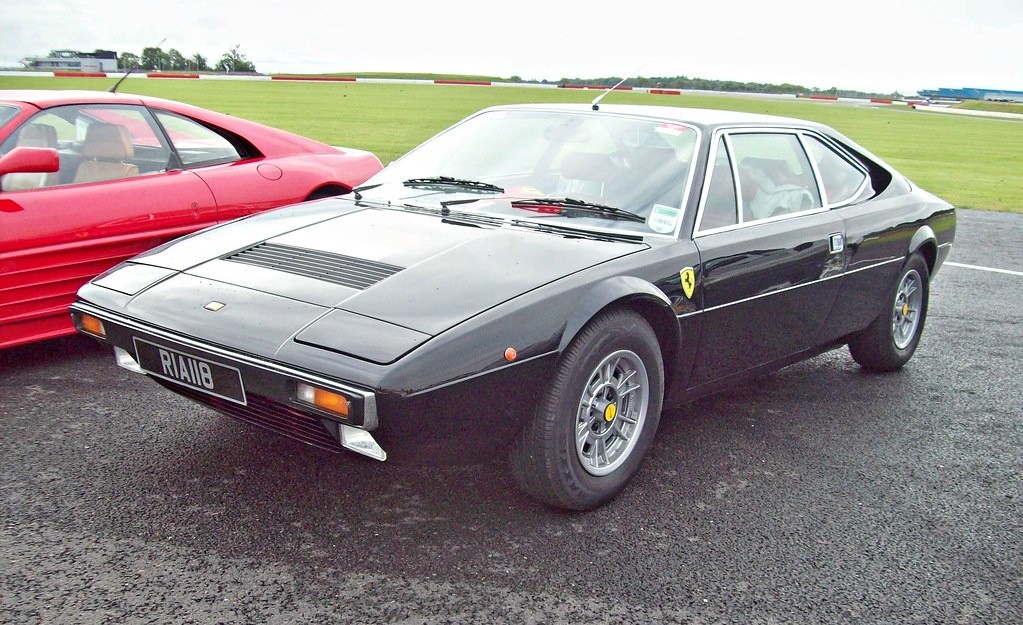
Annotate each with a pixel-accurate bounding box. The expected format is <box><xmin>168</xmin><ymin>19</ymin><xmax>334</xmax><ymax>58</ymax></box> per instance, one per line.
<box><xmin>0</xmin><ymin>90</ymin><xmax>385</xmax><ymax>353</ymax></box>
<box><xmin>68</xmin><ymin>104</ymin><xmax>957</xmax><ymax>511</ymax></box>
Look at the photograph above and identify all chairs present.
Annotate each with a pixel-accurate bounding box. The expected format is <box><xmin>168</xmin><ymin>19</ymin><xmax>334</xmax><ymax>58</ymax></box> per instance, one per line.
<box><xmin>740</xmin><ymin>155</ymin><xmax>789</xmax><ymax>189</ymax></box>
<box><xmin>697</xmin><ymin>163</ymin><xmax>756</xmax><ymax>232</ymax></box>
<box><xmin>1</xmin><ymin>122</ymin><xmax>64</xmax><ymax>192</ymax></box>
<box><xmin>640</xmin><ymin>146</ymin><xmax>680</xmax><ymax>181</ymax></box>
<box><xmin>559</xmin><ymin>152</ymin><xmax>625</xmax><ymax>209</ymax></box>
<box><xmin>73</xmin><ymin>123</ymin><xmax>140</xmax><ymax>186</ymax></box>
